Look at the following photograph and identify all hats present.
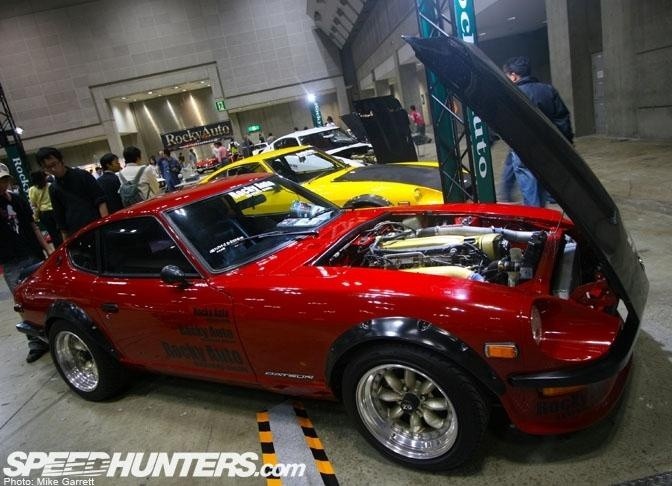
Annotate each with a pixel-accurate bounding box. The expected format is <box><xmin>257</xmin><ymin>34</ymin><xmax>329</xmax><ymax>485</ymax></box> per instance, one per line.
<box><xmin>0</xmin><ymin>163</ymin><xmax>11</xmax><ymax>180</ymax></box>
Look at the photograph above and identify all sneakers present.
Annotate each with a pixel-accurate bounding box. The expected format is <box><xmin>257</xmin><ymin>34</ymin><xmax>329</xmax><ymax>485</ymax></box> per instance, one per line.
<box><xmin>26</xmin><ymin>348</ymin><xmax>50</xmax><ymax>362</ymax></box>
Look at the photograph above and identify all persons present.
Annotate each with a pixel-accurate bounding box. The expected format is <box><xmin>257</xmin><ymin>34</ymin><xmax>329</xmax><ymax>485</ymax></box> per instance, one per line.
<box><xmin>210</xmin><ymin>114</ymin><xmax>336</xmax><ymax>168</ymax></box>
<box><xmin>0</xmin><ymin>162</ymin><xmax>57</xmax><ymax>365</ymax></box>
<box><xmin>35</xmin><ymin>145</ymin><xmax>109</xmax><ymax>244</ymax></box>
<box><xmin>41</xmin><ymin>143</ymin><xmax>202</xmax><ymax>215</ymax></box>
<box><xmin>499</xmin><ymin>152</ymin><xmax>518</xmax><ymax>205</ymax></box>
<box><xmin>26</xmin><ymin>165</ymin><xmax>69</xmax><ymax>250</ymax></box>
<box><xmin>500</xmin><ymin>54</ymin><xmax>575</xmax><ymax>208</ymax></box>
<box><xmin>407</xmin><ymin>105</ymin><xmax>426</xmax><ymax>145</ymax></box>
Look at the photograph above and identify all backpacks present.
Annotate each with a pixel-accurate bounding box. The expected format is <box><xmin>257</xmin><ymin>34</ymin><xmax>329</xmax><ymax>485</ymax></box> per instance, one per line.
<box><xmin>118</xmin><ymin>167</ymin><xmax>148</xmax><ymax>208</ymax></box>
